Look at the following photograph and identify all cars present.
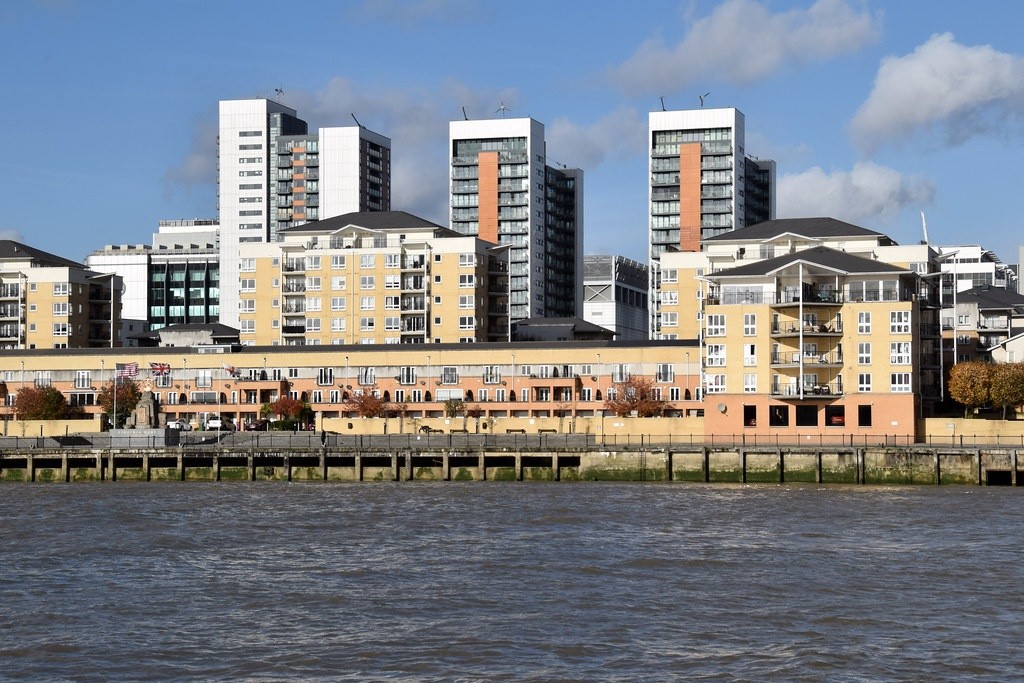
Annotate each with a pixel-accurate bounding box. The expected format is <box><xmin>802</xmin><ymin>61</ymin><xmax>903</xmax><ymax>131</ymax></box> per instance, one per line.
<box><xmin>208</xmin><ymin>415</ymin><xmax>237</xmax><ymax>431</ymax></box>
<box><xmin>246</xmin><ymin>419</ymin><xmax>271</xmax><ymax>431</ymax></box>
<box><xmin>830</xmin><ymin>410</ymin><xmax>844</xmax><ymax>425</ymax></box>
<box><xmin>166</xmin><ymin>418</ymin><xmax>193</xmax><ymax>431</ymax></box>
